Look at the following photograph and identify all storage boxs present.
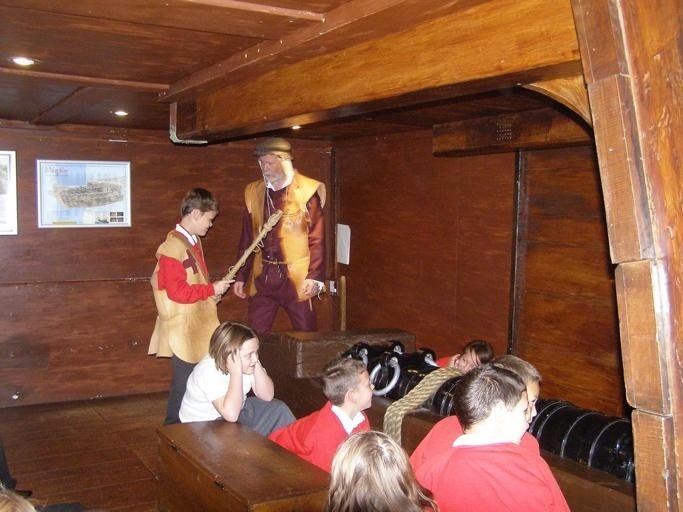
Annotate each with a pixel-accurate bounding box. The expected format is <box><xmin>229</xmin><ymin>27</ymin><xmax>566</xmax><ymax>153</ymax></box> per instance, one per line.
<box><xmin>255</xmin><ymin>327</ymin><xmax>416</xmax><ymax>384</ymax></box>
<box><xmin>157</xmin><ymin>417</ymin><xmax>331</xmax><ymax>512</ymax></box>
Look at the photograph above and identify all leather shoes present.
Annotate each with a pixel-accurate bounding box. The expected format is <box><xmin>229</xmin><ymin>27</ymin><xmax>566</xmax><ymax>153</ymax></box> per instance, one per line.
<box><xmin>14</xmin><ymin>489</ymin><xmax>33</xmax><ymax>499</ymax></box>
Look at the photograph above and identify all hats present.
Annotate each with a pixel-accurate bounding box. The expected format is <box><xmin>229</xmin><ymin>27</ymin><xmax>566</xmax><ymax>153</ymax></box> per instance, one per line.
<box><xmin>252</xmin><ymin>136</ymin><xmax>296</xmax><ymax>161</ymax></box>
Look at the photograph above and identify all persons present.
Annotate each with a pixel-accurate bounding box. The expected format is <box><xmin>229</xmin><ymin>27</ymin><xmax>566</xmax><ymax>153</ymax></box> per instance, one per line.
<box><xmin>179</xmin><ymin>319</ymin><xmax>297</xmax><ymax>437</ymax></box>
<box><xmin>436</xmin><ymin>338</ymin><xmax>496</xmax><ymax>371</ymax></box>
<box><xmin>265</xmin><ymin>356</ymin><xmax>375</xmax><ymax>475</ymax></box>
<box><xmin>148</xmin><ymin>188</ymin><xmax>236</xmax><ymax>426</ymax></box>
<box><xmin>231</xmin><ymin>136</ymin><xmax>327</xmax><ymax>336</ymax></box>
<box><xmin>0</xmin><ymin>434</ymin><xmax>32</xmax><ymax>499</ymax></box>
<box><xmin>322</xmin><ymin>426</ymin><xmax>440</xmax><ymax>511</ymax></box>
<box><xmin>410</xmin><ymin>353</ymin><xmax>544</xmax><ymax>512</ymax></box>
<box><xmin>434</xmin><ymin>364</ymin><xmax>572</xmax><ymax>512</ymax></box>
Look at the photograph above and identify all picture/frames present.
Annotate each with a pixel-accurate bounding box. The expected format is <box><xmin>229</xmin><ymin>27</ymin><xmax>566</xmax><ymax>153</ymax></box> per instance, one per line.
<box><xmin>36</xmin><ymin>159</ymin><xmax>132</xmax><ymax>229</ymax></box>
<box><xmin>0</xmin><ymin>150</ymin><xmax>17</xmax><ymax>236</ymax></box>
